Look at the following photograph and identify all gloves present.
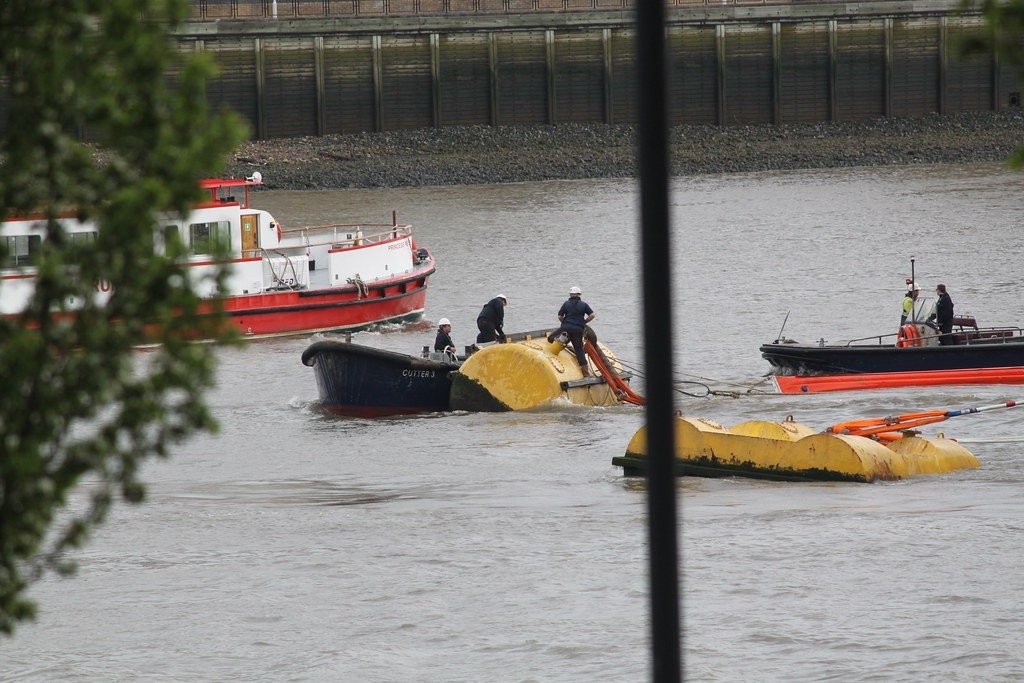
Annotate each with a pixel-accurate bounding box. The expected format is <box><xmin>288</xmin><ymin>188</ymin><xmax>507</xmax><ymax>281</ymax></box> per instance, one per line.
<box><xmin>495</xmin><ymin>321</ymin><xmax>504</xmax><ymax>330</ymax></box>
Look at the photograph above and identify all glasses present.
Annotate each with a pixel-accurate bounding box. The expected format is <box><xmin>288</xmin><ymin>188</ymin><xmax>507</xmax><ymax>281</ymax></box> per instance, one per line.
<box><xmin>915</xmin><ymin>290</ymin><xmax>918</xmax><ymax>292</ymax></box>
<box><xmin>448</xmin><ymin>325</ymin><xmax>450</xmax><ymax>326</ymax></box>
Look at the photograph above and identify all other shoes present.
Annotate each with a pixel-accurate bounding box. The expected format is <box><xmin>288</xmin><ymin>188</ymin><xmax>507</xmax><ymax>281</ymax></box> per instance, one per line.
<box><xmin>547</xmin><ymin>337</ymin><xmax>553</xmax><ymax>343</ymax></box>
<box><xmin>582</xmin><ymin>366</ymin><xmax>590</xmax><ymax>377</ymax></box>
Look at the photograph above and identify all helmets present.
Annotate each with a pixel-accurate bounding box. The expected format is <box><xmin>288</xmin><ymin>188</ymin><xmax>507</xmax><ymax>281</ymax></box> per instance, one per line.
<box><xmin>497</xmin><ymin>294</ymin><xmax>508</xmax><ymax>306</ymax></box>
<box><xmin>907</xmin><ymin>283</ymin><xmax>921</xmax><ymax>291</ymax></box>
<box><xmin>569</xmin><ymin>287</ymin><xmax>581</xmax><ymax>294</ymax></box>
<box><xmin>439</xmin><ymin>318</ymin><xmax>451</xmax><ymax>325</ymax></box>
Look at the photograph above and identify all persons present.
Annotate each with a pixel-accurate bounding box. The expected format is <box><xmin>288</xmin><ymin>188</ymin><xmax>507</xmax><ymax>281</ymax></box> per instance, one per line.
<box><xmin>434</xmin><ymin>318</ymin><xmax>458</xmax><ymax>360</ymax></box>
<box><xmin>547</xmin><ymin>286</ymin><xmax>597</xmax><ymax>377</ymax></box>
<box><xmin>936</xmin><ymin>284</ymin><xmax>954</xmax><ymax>333</ymax></box>
<box><xmin>901</xmin><ymin>280</ymin><xmax>921</xmax><ymax>326</ymax></box>
<box><xmin>476</xmin><ymin>294</ymin><xmax>508</xmax><ymax>344</ymax></box>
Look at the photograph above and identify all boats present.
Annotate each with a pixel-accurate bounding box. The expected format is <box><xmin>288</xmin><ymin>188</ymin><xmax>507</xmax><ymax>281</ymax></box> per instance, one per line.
<box><xmin>0</xmin><ymin>170</ymin><xmax>440</xmax><ymax>354</ymax></box>
<box><xmin>612</xmin><ymin>409</ymin><xmax>982</xmax><ymax>483</ymax></box>
<box><xmin>758</xmin><ymin>255</ymin><xmax>1024</xmax><ymax>373</ymax></box>
<box><xmin>300</xmin><ymin>325</ymin><xmax>589</xmax><ymax>419</ymax></box>
<box><xmin>774</xmin><ymin>365</ymin><xmax>1024</xmax><ymax>394</ymax></box>
